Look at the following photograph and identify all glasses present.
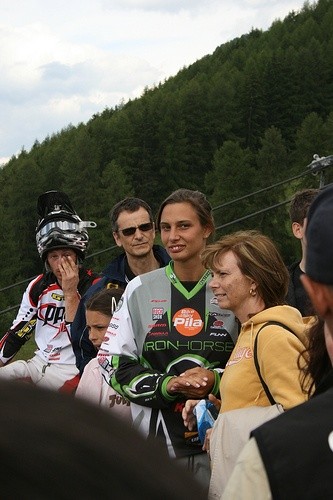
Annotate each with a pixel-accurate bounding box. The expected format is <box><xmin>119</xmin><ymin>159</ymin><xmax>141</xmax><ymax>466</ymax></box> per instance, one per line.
<box><xmin>116</xmin><ymin>222</ymin><xmax>154</xmax><ymax>236</ymax></box>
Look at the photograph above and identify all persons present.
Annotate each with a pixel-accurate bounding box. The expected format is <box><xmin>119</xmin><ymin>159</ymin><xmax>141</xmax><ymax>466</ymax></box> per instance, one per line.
<box><xmin>200</xmin><ymin>190</ymin><xmax>333</xmax><ymax>500</ymax></box>
<box><xmin>0</xmin><ymin>191</ymin><xmax>106</xmax><ymax>397</ymax></box>
<box><xmin>96</xmin><ymin>189</ymin><xmax>242</xmax><ymax>490</ymax></box>
<box><xmin>0</xmin><ymin>380</ymin><xmax>209</xmax><ymax>500</ymax></box>
<box><xmin>70</xmin><ymin>198</ymin><xmax>169</xmax><ymax>377</ymax></box>
<box><xmin>75</xmin><ymin>289</ymin><xmax>133</xmax><ymax>423</ymax></box>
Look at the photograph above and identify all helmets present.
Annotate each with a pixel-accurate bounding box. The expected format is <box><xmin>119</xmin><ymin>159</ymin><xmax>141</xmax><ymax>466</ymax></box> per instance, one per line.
<box><xmin>34</xmin><ymin>191</ymin><xmax>97</xmax><ymax>260</ymax></box>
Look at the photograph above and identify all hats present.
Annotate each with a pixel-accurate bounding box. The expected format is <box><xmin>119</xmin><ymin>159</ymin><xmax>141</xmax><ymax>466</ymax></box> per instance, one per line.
<box><xmin>304</xmin><ymin>183</ymin><xmax>333</xmax><ymax>286</ymax></box>
